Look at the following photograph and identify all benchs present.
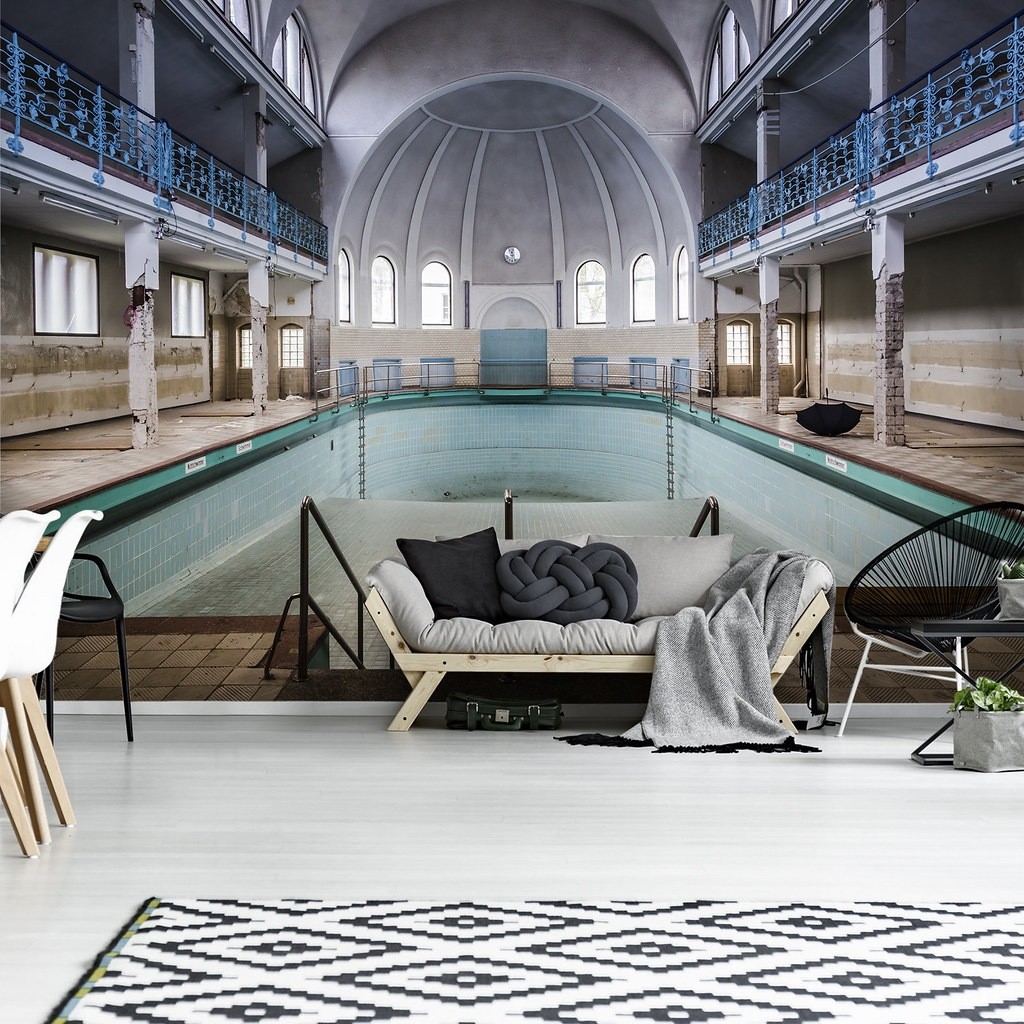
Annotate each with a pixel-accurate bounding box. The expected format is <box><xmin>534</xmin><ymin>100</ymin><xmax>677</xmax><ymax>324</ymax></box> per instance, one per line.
<box><xmin>359</xmin><ymin>547</ymin><xmax>839</xmax><ymax>752</ymax></box>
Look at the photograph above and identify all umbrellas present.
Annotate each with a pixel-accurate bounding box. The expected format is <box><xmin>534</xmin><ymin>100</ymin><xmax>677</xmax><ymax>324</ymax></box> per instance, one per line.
<box><xmin>794</xmin><ymin>388</ymin><xmax>864</xmax><ymax>437</ymax></box>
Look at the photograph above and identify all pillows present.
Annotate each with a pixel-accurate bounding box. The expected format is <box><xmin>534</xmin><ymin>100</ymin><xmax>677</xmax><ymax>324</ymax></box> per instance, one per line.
<box><xmin>394</xmin><ymin>526</ymin><xmax>510</xmax><ymax>621</ymax></box>
<box><xmin>585</xmin><ymin>532</ymin><xmax>736</xmax><ymax>614</ymax></box>
<box><xmin>432</xmin><ymin>532</ymin><xmax>589</xmax><ymax>555</ymax></box>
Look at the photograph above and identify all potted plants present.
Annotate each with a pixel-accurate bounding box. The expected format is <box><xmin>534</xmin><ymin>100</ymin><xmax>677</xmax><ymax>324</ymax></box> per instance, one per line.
<box><xmin>952</xmin><ymin>674</ymin><xmax>1024</xmax><ymax>774</ymax></box>
<box><xmin>998</xmin><ymin>561</ymin><xmax>1024</xmax><ymax>621</ymax></box>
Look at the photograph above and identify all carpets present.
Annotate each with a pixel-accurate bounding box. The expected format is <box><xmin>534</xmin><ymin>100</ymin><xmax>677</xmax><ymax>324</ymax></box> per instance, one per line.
<box><xmin>46</xmin><ymin>890</ymin><xmax>1024</xmax><ymax>1024</ymax></box>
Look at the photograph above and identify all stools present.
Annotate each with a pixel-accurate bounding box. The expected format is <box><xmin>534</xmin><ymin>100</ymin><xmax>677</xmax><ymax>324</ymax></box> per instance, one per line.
<box><xmin>27</xmin><ymin>549</ymin><xmax>133</xmax><ymax>745</ymax></box>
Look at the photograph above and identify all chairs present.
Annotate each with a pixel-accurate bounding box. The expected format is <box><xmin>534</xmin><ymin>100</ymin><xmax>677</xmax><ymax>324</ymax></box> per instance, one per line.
<box><xmin>834</xmin><ymin>501</ymin><xmax>1024</xmax><ymax>738</ymax></box>
<box><xmin>0</xmin><ymin>509</ymin><xmax>60</xmax><ymax>862</ymax></box>
<box><xmin>17</xmin><ymin>510</ymin><xmax>108</xmax><ymax>832</ymax></box>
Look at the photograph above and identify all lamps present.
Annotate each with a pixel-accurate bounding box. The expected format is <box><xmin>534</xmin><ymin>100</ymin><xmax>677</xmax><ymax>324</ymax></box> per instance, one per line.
<box><xmin>210</xmin><ymin>44</ymin><xmax>246</xmax><ymax>84</ymax></box>
<box><xmin>820</xmin><ymin>225</ymin><xmax>867</xmax><ymax>247</ymax></box>
<box><xmin>737</xmin><ymin>263</ymin><xmax>757</xmax><ymax>274</ymax></box>
<box><xmin>713</xmin><ymin>269</ymin><xmax>735</xmax><ymax>282</ymax></box>
<box><xmin>293</xmin><ymin>274</ymin><xmax>314</xmax><ymax>284</ymax></box>
<box><xmin>157</xmin><ymin>230</ymin><xmax>206</xmax><ymax>253</ymax></box>
<box><xmin>292</xmin><ymin>126</ymin><xmax>313</xmax><ymax>149</ymax></box>
<box><xmin>778</xmin><ymin>242</ymin><xmax>815</xmax><ymax>260</ymax></box>
<box><xmin>214</xmin><ymin>251</ymin><xmax>248</xmax><ymax>265</ymax></box>
<box><xmin>819</xmin><ymin>0</ymin><xmax>853</xmax><ymax>35</ymax></box>
<box><xmin>710</xmin><ymin>121</ymin><xmax>731</xmax><ymax>144</ymax></box>
<box><xmin>0</xmin><ymin>178</ymin><xmax>20</xmax><ymax>196</ymax></box>
<box><xmin>776</xmin><ymin>37</ymin><xmax>814</xmax><ymax>78</ymax></box>
<box><xmin>1011</xmin><ymin>174</ymin><xmax>1024</xmax><ymax>186</ymax></box>
<box><xmin>266</xmin><ymin>99</ymin><xmax>291</xmax><ymax>126</ymax></box>
<box><xmin>732</xmin><ymin>94</ymin><xmax>757</xmax><ymax>121</ymax></box>
<box><xmin>161</xmin><ymin>0</ymin><xmax>205</xmax><ymax>42</ymax></box>
<box><xmin>272</xmin><ymin>268</ymin><xmax>291</xmax><ymax>278</ymax></box>
<box><xmin>39</xmin><ymin>191</ymin><xmax>120</xmax><ymax>226</ymax></box>
<box><xmin>908</xmin><ymin>182</ymin><xmax>994</xmax><ymax>220</ymax></box>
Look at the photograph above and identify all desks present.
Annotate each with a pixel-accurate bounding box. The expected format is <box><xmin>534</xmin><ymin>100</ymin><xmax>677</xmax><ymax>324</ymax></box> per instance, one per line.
<box><xmin>908</xmin><ymin>621</ymin><xmax>1024</xmax><ymax>766</ymax></box>
